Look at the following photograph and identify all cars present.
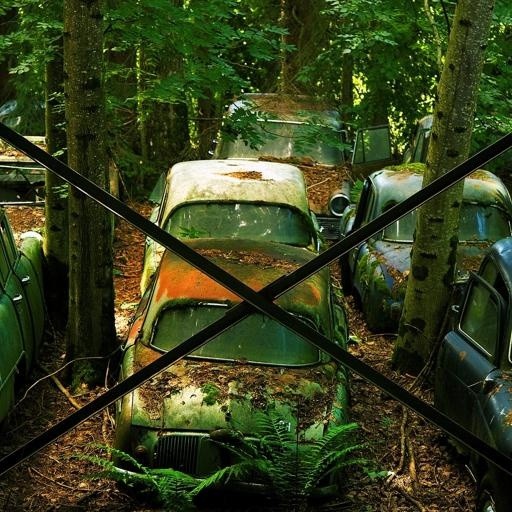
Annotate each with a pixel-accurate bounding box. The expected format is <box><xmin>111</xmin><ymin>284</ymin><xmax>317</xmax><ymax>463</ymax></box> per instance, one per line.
<box><xmin>340</xmin><ymin>163</ymin><xmax>512</xmax><ymax>331</ymax></box>
<box><xmin>434</xmin><ymin>237</ymin><xmax>511</xmax><ymax>497</ymax></box>
<box><xmin>0</xmin><ymin>206</ymin><xmax>46</xmax><ymax>431</ymax></box>
<box><xmin>141</xmin><ymin>156</ymin><xmax>321</xmax><ymax>296</ymax></box>
<box><xmin>109</xmin><ymin>238</ymin><xmax>350</xmax><ymax>500</ymax></box>
<box><xmin>209</xmin><ymin>93</ymin><xmax>392</xmax><ymax>247</ymax></box>
<box><xmin>0</xmin><ymin>135</ymin><xmax>117</xmax><ymax>257</ymax></box>
<box><xmin>404</xmin><ymin>114</ymin><xmax>512</xmax><ymax>199</ymax></box>
